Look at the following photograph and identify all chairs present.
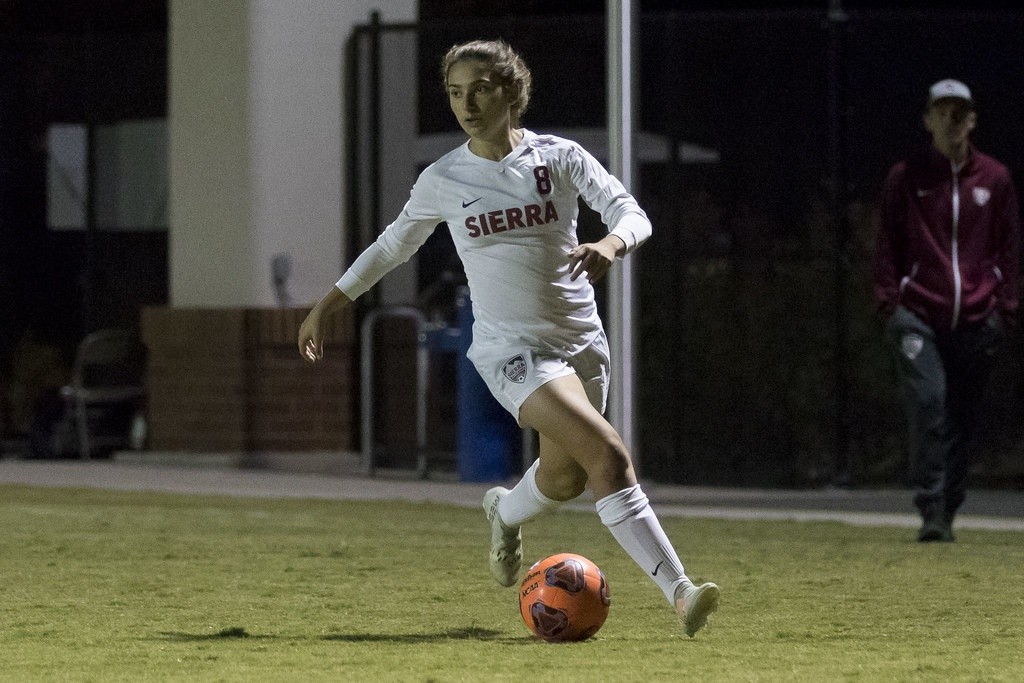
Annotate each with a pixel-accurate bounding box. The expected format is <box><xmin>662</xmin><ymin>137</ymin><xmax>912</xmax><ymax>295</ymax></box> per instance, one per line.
<box><xmin>50</xmin><ymin>328</ymin><xmax>146</xmax><ymax>461</ymax></box>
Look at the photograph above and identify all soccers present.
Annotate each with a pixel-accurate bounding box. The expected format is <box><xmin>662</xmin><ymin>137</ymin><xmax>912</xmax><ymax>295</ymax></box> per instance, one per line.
<box><xmin>517</xmin><ymin>551</ymin><xmax>611</xmax><ymax>644</ymax></box>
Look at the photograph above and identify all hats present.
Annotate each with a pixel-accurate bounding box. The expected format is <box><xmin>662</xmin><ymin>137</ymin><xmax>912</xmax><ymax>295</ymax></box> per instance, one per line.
<box><xmin>928</xmin><ymin>78</ymin><xmax>974</xmax><ymax>106</ymax></box>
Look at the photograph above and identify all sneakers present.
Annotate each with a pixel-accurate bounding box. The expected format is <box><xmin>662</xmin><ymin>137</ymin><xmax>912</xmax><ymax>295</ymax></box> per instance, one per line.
<box><xmin>482</xmin><ymin>486</ymin><xmax>523</xmax><ymax>587</ymax></box>
<box><xmin>673</xmin><ymin>580</ymin><xmax>720</xmax><ymax>638</ymax></box>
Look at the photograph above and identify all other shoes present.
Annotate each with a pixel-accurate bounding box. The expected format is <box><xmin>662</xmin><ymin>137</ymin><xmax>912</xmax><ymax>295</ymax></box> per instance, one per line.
<box><xmin>917</xmin><ymin>518</ymin><xmax>955</xmax><ymax>542</ymax></box>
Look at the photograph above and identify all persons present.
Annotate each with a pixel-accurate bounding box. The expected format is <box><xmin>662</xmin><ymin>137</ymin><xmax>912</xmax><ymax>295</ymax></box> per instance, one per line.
<box><xmin>411</xmin><ymin>255</ymin><xmax>513</xmax><ymax>482</ymax></box>
<box><xmin>875</xmin><ymin>80</ymin><xmax>1024</xmax><ymax>543</ymax></box>
<box><xmin>298</xmin><ymin>41</ymin><xmax>720</xmax><ymax>638</ymax></box>
<box><xmin>23</xmin><ymin>271</ymin><xmax>146</xmax><ymax>458</ymax></box>
